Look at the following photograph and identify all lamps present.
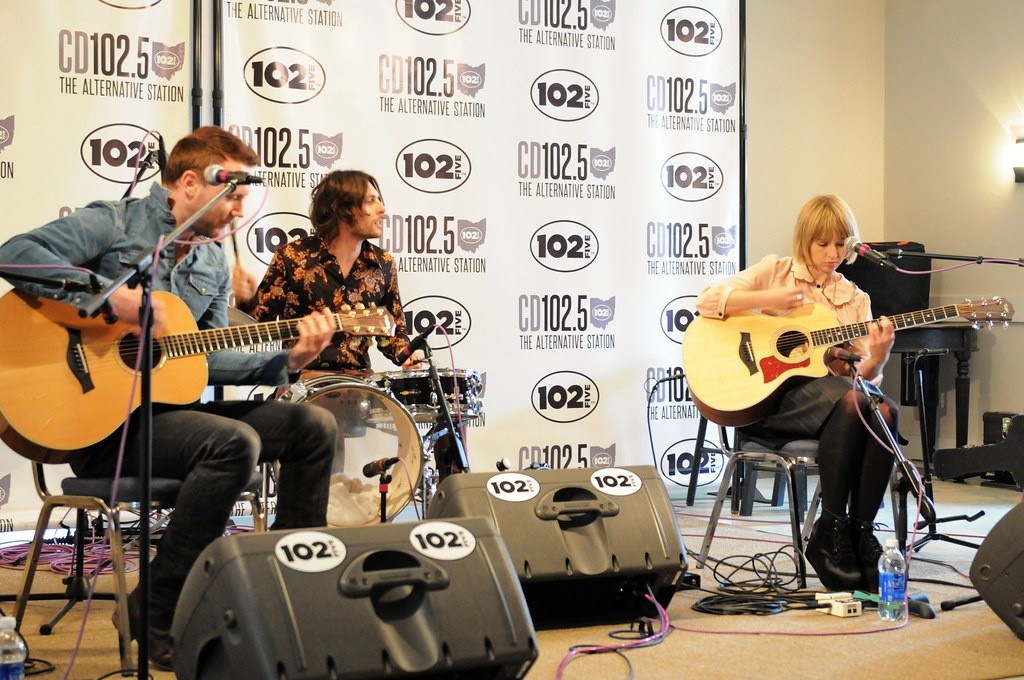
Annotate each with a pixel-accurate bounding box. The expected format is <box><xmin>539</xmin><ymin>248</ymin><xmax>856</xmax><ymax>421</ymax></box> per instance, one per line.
<box><xmin>1013</xmin><ymin>137</ymin><xmax>1024</xmax><ymax>184</ymax></box>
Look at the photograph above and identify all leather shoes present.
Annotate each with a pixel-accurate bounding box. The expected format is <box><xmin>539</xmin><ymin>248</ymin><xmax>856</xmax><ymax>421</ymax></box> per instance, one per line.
<box><xmin>110</xmin><ymin>592</ymin><xmax>178</xmax><ymax>672</ymax></box>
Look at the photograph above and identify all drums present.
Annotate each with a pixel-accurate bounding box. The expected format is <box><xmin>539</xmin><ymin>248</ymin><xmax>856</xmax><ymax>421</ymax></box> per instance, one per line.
<box><xmin>373</xmin><ymin>368</ymin><xmax>483</xmax><ymax>423</ymax></box>
<box><xmin>275</xmin><ymin>373</ymin><xmax>428</xmax><ymax>531</ymax></box>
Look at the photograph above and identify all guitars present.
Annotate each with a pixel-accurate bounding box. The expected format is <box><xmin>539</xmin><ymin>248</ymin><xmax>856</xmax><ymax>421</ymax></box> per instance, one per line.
<box><xmin>681</xmin><ymin>294</ymin><xmax>1018</xmax><ymax>431</ymax></box>
<box><xmin>0</xmin><ymin>284</ymin><xmax>400</xmax><ymax>466</ymax></box>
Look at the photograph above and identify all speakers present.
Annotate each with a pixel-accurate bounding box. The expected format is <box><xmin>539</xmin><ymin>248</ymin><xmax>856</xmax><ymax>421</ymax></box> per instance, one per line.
<box><xmin>167</xmin><ymin>516</ymin><xmax>540</xmax><ymax>680</ymax></box>
<box><xmin>425</xmin><ymin>463</ymin><xmax>688</xmax><ymax>631</ymax></box>
<box><xmin>968</xmin><ymin>499</ymin><xmax>1024</xmax><ymax>641</ymax></box>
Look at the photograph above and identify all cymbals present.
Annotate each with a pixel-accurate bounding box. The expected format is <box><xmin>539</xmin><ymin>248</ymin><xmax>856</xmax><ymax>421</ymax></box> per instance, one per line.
<box><xmin>227</xmin><ymin>305</ymin><xmax>259</xmax><ymax>327</ymax></box>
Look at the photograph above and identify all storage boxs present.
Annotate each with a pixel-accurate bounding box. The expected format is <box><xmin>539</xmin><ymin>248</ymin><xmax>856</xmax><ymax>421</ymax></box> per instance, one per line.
<box><xmin>983</xmin><ymin>411</ymin><xmax>1022</xmax><ymax>486</ymax></box>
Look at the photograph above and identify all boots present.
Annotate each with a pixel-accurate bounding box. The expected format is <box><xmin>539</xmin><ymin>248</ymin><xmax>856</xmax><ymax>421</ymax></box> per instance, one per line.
<box><xmin>803</xmin><ymin>493</ymin><xmax>863</xmax><ymax>593</ymax></box>
<box><xmin>848</xmin><ymin>516</ymin><xmax>884</xmax><ymax>595</ymax></box>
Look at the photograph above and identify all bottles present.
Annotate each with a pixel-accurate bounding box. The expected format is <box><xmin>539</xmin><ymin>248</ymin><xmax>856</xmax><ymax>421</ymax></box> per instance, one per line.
<box><xmin>878</xmin><ymin>540</ymin><xmax>907</xmax><ymax>621</ymax></box>
<box><xmin>0</xmin><ymin>616</ymin><xmax>27</xmax><ymax>680</ymax></box>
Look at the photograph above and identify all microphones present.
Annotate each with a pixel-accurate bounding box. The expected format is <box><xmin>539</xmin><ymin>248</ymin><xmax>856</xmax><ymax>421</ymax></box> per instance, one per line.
<box><xmin>828</xmin><ymin>347</ymin><xmax>862</xmax><ymax>362</ymax></box>
<box><xmin>203</xmin><ymin>164</ymin><xmax>263</xmax><ymax>186</ymax></box>
<box><xmin>158</xmin><ymin>135</ymin><xmax>165</xmax><ymax>181</ymax></box>
<box><xmin>363</xmin><ymin>456</ymin><xmax>399</xmax><ymax>478</ymax></box>
<box><xmin>394</xmin><ymin>325</ymin><xmax>435</xmax><ymax>366</ymax></box>
<box><xmin>845</xmin><ymin>236</ymin><xmax>899</xmax><ymax>271</ymax></box>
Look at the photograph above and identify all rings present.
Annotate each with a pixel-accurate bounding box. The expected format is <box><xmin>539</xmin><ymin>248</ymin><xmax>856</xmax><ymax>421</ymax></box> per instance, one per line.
<box><xmin>797</xmin><ymin>294</ymin><xmax>803</xmax><ymax>300</ymax></box>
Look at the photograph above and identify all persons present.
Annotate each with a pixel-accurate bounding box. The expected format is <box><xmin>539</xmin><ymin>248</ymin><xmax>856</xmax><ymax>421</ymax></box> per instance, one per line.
<box><xmin>1</xmin><ymin>125</ymin><xmax>337</xmax><ymax>670</ymax></box>
<box><xmin>696</xmin><ymin>194</ymin><xmax>899</xmax><ymax>591</ymax></box>
<box><xmin>233</xmin><ymin>169</ymin><xmax>422</xmax><ymax>472</ymax></box>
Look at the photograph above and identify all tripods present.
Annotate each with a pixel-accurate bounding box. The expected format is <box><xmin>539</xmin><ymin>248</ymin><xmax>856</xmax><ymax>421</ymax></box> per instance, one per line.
<box><xmin>906</xmin><ymin>349</ymin><xmax>981</xmax><ymax>554</ymax></box>
<box><xmin>0</xmin><ymin>150</ymin><xmax>159</xmax><ymax>633</ymax></box>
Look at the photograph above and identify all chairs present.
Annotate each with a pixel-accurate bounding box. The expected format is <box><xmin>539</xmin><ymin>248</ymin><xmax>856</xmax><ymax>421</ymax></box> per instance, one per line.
<box><xmin>694</xmin><ymin>424</ymin><xmax>898</xmax><ymax>591</ymax></box>
<box><xmin>12</xmin><ymin>458</ymin><xmax>265</xmax><ymax>677</ymax></box>
